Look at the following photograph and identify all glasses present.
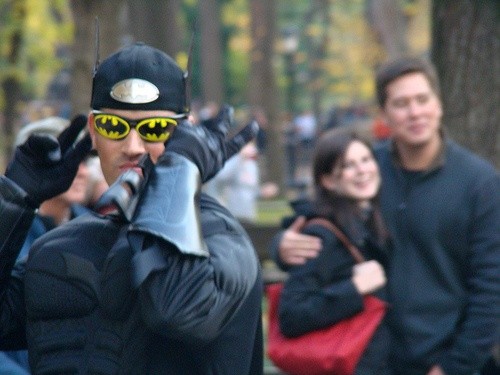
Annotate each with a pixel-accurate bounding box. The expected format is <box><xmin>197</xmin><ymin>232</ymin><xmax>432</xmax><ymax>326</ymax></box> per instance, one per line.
<box><xmin>90</xmin><ymin>111</ymin><xmax>187</xmax><ymax>144</ymax></box>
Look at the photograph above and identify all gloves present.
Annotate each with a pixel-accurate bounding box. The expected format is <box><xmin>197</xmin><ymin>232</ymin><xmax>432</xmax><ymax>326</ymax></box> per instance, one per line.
<box><xmin>165</xmin><ymin>106</ymin><xmax>263</xmax><ymax>181</ymax></box>
<box><xmin>5</xmin><ymin>115</ymin><xmax>92</xmax><ymax>205</ymax></box>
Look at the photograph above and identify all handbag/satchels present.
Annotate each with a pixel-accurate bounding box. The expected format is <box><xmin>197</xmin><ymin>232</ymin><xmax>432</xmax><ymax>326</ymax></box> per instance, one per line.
<box><xmin>266</xmin><ymin>217</ymin><xmax>386</xmax><ymax>370</ymax></box>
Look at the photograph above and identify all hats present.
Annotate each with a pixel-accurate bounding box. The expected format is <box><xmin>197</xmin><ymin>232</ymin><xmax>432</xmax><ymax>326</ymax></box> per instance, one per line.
<box><xmin>92</xmin><ymin>12</ymin><xmax>193</xmax><ymax>113</ymax></box>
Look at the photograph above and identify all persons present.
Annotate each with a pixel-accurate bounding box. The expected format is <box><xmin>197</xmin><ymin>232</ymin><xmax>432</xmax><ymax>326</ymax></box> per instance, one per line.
<box><xmin>181</xmin><ymin>99</ymin><xmax>378</xmax><ymax>264</ymax></box>
<box><xmin>0</xmin><ymin>16</ymin><xmax>263</xmax><ymax>375</ymax></box>
<box><xmin>268</xmin><ymin>54</ymin><xmax>500</xmax><ymax>375</ymax></box>
<box><xmin>0</xmin><ymin>116</ymin><xmax>116</xmax><ymax>375</ymax></box>
<box><xmin>276</xmin><ymin>126</ymin><xmax>400</xmax><ymax>375</ymax></box>
